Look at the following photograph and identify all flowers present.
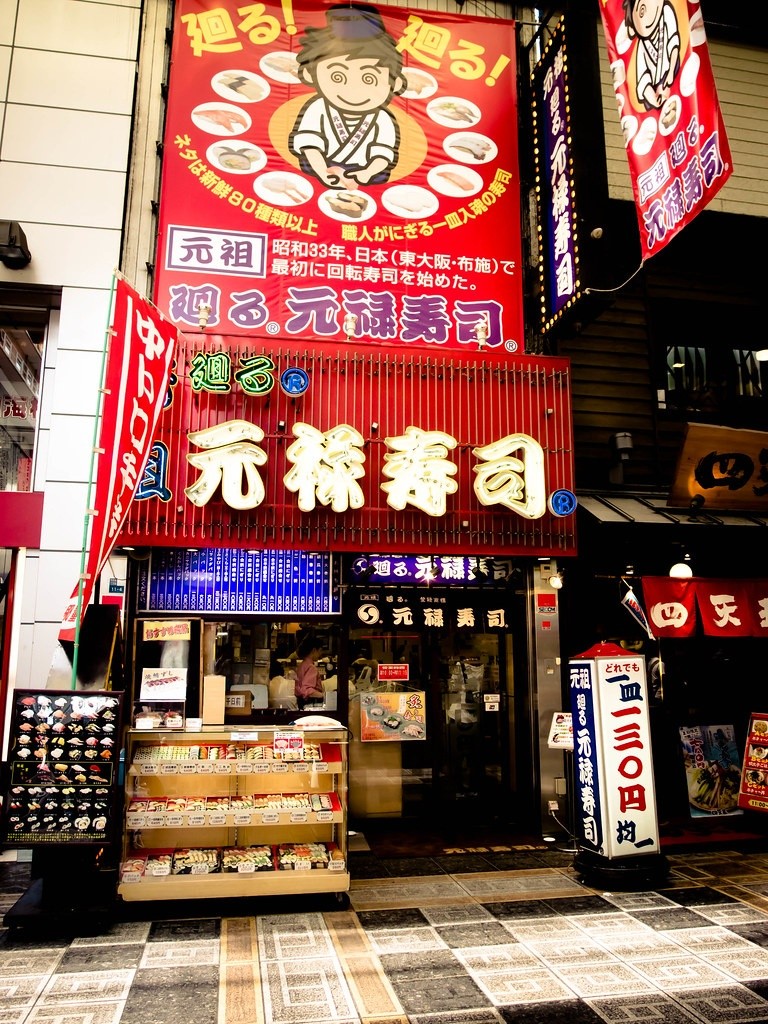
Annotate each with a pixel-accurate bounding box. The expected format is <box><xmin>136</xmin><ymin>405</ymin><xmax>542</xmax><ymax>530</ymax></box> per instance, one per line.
<box><xmin>165</xmin><ymin>711</ymin><xmax>179</xmax><ymax>718</ymax></box>
<box><xmin>136</xmin><ymin>712</ymin><xmax>146</xmax><ymax>718</ymax></box>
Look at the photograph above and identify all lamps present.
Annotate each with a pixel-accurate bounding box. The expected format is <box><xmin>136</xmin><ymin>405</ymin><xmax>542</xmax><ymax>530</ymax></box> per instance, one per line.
<box><xmin>547</xmin><ymin>572</ymin><xmax>564</xmax><ymax>589</ymax></box>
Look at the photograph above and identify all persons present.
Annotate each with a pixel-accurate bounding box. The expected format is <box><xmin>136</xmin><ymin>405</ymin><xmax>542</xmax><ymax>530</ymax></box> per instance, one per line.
<box><xmin>450</xmin><ymin>634</ymin><xmax>499</xmax><ymax>798</ymax></box>
<box><xmin>268</xmin><ymin>659</ymin><xmax>297</xmax><ymax>711</ymax></box>
<box><xmin>297</xmin><ymin>638</ymin><xmax>325</xmax><ymax>711</ymax></box>
<box><xmin>320</xmin><ymin>664</ymin><xmax>357</xmax><ymax>712</ymax></box>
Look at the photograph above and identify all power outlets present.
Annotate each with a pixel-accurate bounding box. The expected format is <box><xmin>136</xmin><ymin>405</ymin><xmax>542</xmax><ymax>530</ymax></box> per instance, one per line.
<box><xmin>548</xmin><ymin>800</ymin><xmax>560</xmax><ymax>811</ymax></box>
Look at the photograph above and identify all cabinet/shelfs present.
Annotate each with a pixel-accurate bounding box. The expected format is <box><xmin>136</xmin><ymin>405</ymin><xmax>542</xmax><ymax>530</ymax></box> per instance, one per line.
<box><xmin>117</xmin><ymin>723</ymin><xmax>351</xmax><ymax>911</ymax></box>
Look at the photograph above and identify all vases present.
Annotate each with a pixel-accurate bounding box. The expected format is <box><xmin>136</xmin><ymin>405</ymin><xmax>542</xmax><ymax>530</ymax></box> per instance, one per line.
<box><xmin>165</xmin><ymin>716</ymin><xmax>183</xmax><ymax>727</ymax></box>
<box><xmin>136</xmin><ymin>718</ymin><xmax>154</xmax><ymax>729</ymax></box>
<box><xmin>147</xmin><ymin>716</ymin><xmax>161</xmax><ymax>727</ymax></box>
